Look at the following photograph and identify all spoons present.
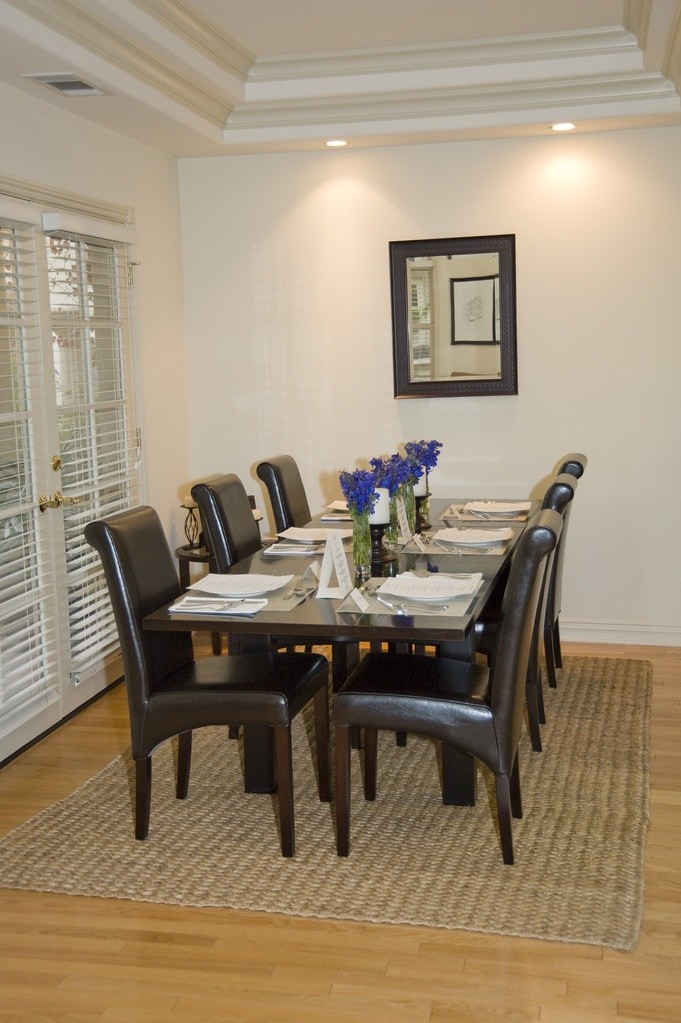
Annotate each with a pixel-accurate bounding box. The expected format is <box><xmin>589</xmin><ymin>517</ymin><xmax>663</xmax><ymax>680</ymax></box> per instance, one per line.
<box><xmin>176</xmin><ymin>602</ymin><xmax>231</xmax><ymax>611</ymax></box>
<box><xmin>417</xmin><ymin>569</ymin><xmax>472</xmax><ymax>576</ymax></box>
<box><xmin>411</xmin><ymin>570</ymin><xmax>472</xmax><ymax>578</ymax></box>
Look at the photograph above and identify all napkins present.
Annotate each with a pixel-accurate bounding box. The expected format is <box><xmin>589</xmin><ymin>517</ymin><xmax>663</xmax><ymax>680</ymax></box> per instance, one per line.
<box><xmin>320</xmin><ymin>513</ymin><xmax>353</xmax><ymax>520</ymax></box>
<box><xmin>395</xmin><ymin>571</ymin><xmax>482</xmax><ymax>580</ymax></box>
<box><xmin>263</xmin><ymin>544</ymin><xmax>325</xmax><ymax>554</ymax></box>
<box><xmin>167</xmin><ymin>596</ymin><xmax>267</xmax><ymax>614</ymax></box>
<box><xmin>445</xmin><ymin>528</ymin><xmax>511</xmax><ymax>531</ymax></box>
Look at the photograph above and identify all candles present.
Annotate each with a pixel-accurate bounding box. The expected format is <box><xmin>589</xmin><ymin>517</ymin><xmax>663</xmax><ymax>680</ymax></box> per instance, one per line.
<box><xmin>367</xmin><ymin>488</ymin><xmax>390</xmax><ymax>524</ymax></box>
<box><xmin>412</xmin><ymin>466</ymin><xmax>426</xmax><ymax>496</ymax></box>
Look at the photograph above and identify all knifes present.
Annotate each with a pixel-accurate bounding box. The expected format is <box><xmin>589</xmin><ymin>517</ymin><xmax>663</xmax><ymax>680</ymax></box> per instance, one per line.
<box><xmin>186</xmin><ymin>599</ymin><xmax>264</xmax><ymax>604</ymax></box>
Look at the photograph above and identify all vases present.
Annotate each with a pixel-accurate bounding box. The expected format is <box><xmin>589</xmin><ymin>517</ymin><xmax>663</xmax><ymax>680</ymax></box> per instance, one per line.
<box><xmin>382</xmin><ymin>496</ymin><xmax>399</xmax><ymax>553</ymax></box>
<box><xmin>397</xmin><ymin>485</ymin><xmax>416</xmax><ymax>535</ymax></box>
<box><xmin>351</xmin><ymin>513</ymin><xmax>373</xmax><ymax>575</ymax></box>
<box><xmin>418</xmin><ymin>474</ymin><xmax>430</xmax><ymax>520</ymax></box>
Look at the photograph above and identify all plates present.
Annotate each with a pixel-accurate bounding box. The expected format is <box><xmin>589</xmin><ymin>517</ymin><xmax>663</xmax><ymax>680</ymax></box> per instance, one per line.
<box><xmin>479</xmin><ymin>512</ymin><xmax>516</xmax><ymax>516</ymax></box>
<box><xmin>200</xmin><ymin>590</ymin><xmax>267</xmax><ymax>597</ymax></box>
<box><xmin>450</xmin><ymin>540</ymin><xmax>492</xmax><ymax>546</ymax></box>
<box><xmin>408</xmin><ymin>597</ymin><xmax>453</xmax><ymax>601</ymax></box>
<box><xmin>295</xmin><ymin>539</ymin><xmax>326</xmax><ymax>543</ymax></box>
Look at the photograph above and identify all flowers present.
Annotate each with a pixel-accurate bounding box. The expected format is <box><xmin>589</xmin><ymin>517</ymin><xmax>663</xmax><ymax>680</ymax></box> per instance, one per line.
<box><xmin>339</xmin><ymin>439</ymin><xmax>443</xmax><ymax>513</ymax></box>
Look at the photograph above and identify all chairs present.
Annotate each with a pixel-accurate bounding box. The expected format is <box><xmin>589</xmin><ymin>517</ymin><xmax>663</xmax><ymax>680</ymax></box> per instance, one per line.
<box><xmin>84</xmin><ymin>505</ymin><xmax>334</xmax><ymax>856</ymax></box>
<box><xmin>190</xmin><ymin>473</ymin><xmax>332</xmax><ymax>739</ymax></box>
<box><xmin>332</xmin><ymin>509</ymin><xmax>564</xmax><ymax>866</ymax></box>
<box><xmin>256</xmin><ymin>456</ymin><xmax>312</xmax><ymax>654</ymax></box>
<box><xmin>395</xmin><ymin>473</ymin><xmax>578</xmax><ymax>751</ymax></box>
<box><xmin>470</xmin><ymin>453</ymin><xmax>586</xmax><ymax>689</ymax></box>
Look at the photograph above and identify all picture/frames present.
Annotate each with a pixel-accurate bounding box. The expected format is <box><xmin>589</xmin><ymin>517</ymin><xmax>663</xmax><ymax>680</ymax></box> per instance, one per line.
<box><xmin>450</xmin><ymin>275</ymin><xmax>496</xmax><ymax>345</ymax></box>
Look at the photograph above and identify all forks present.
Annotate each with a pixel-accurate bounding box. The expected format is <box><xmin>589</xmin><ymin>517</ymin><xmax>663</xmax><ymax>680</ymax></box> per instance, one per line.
<box><xmin>180</xmin><ymin>602</ymin><xmax>235</xmax><ymax>607</ymax></box>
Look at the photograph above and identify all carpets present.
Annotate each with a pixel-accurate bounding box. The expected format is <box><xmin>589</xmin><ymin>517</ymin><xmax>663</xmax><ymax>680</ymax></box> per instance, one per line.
<box><xmin>0</xmin><ymin>648</ymin><xmax>653</xmax><ymax>953</ymax></box>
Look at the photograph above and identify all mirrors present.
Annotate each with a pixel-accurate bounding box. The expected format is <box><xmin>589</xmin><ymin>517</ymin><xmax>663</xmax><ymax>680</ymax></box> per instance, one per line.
<box><xmin>388</xmin><ymin>234</ymin><xmax>519</xmax><ymax>399</ymax></box>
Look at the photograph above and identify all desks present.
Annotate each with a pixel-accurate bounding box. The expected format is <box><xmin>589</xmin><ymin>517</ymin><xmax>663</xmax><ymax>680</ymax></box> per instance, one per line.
<box><xmin>174</xmin><ymin>540</ymin><xmax>272</xmax><ymax>655</ymax></box>
<box><xmin>141</xmin><ymin>498</ymin><xmax>542</xmax><ymax>806</ymax></box>
